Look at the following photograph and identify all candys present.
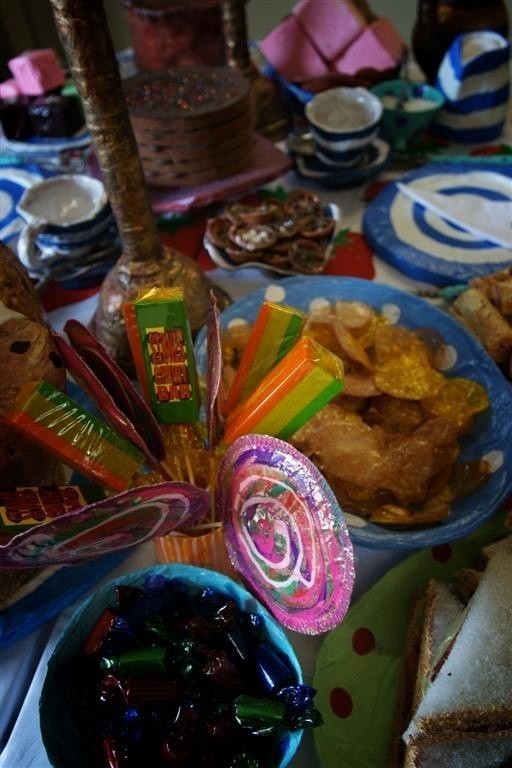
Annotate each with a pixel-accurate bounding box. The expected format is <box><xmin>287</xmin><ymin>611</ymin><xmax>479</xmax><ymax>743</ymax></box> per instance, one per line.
<box><xmin>0</xmin><ymin>186</ymin><xmax>489</xmax><ymax>768</ymax></box>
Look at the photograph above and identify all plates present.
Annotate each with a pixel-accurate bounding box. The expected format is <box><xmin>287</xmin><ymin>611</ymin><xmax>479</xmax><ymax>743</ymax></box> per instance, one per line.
<box><xmin>1</xmin><ymin>161</ymin><xmax>59</xmax><ymax>244</ymax></box>
<box><xmin>0</xmin><ymin>122</ymin><xmax>95</xmax><ymax>155</ymax></box>
<box><xmin>25</xmin><ymin>241</ymin><xmax>122</xmax><ymax>291</ymax></box>
<box><xmin>290</xmin><ymin>127</ymin><xmax>392</xmax><ymax>193</ymax></box>
<box><xmin>310</xmin><ymin>534</ymin><xmax>490</xmax><ymax>767</ymax></box>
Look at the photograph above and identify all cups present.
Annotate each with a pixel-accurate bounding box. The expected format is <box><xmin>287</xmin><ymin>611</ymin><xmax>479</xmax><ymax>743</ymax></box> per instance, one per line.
<box><xmin>368</xmin><ymin>79</ymin><xmax>448</xmax><ymax>167</ymax></box>
<box><xmin>14</xmin><ymin>174</ymin><xmax>113</xmax><ymax>275</ymax></box>
<box><xmin>306</xmin><ymin>86</ymin><xmax>385</xmax><ymax>172</ymax></box>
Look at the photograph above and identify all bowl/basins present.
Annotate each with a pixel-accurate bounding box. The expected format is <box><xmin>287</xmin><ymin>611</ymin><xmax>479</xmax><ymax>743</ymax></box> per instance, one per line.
<box><xmin>200</xmin><ymin>196</ymin><xmax>342</xmax><ymax>278</ymax></box>
<box><xmin>188</xmin><ymin>272</ymin><xmax>511</xmax><ymax>549</ymax></box>
<box><xmin>36</xmin><ymin>559</ymin><xmax>307</xmax><ymax>766</ymax></box>
<box><xmin>118</xmin><ymin>63</ymin><xmax>258</xmax><ymax>188</ymax></box>
